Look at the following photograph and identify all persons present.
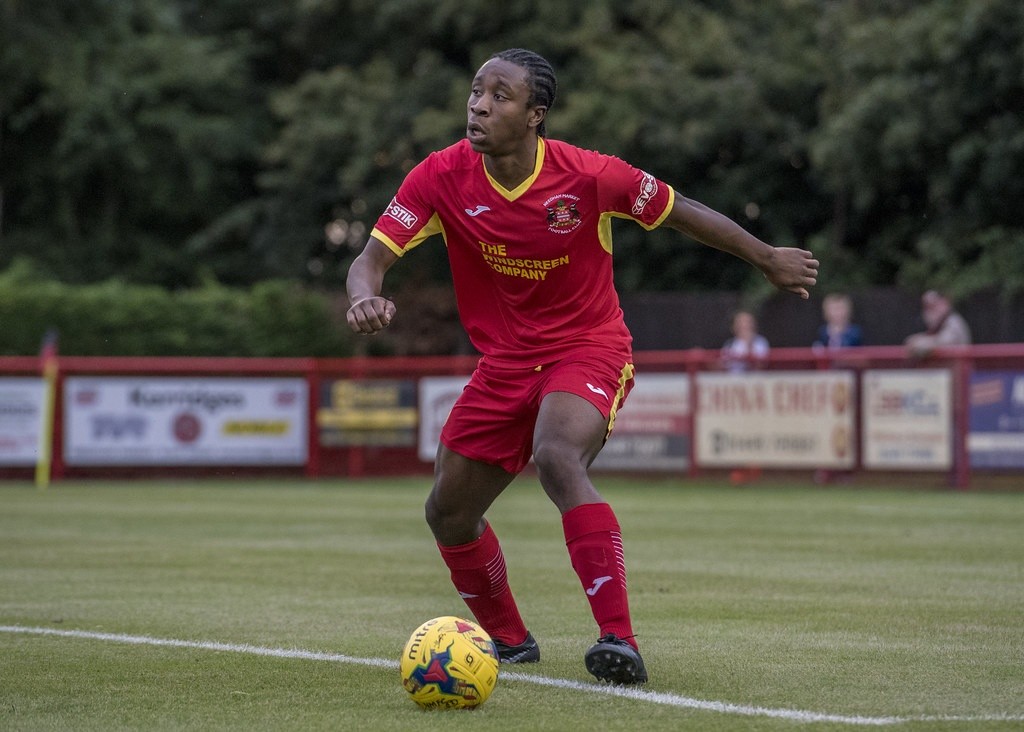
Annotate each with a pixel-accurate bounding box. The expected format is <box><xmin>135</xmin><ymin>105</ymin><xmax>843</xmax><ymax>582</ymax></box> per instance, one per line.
<box><xmin>813</xmin><ymin>292</ymin><xmax>861</xmax><ymax>349</ymax></box>
<box><xmin>346</xmin><ymin>48</ymin><xmax>819</xmax><ymax>684</ymax></box>
<box><xmin>720</xmin><ymin>310</ymin><xmax>770</xmax><ymax>374</ymax></box>
<box><xmin>906</xmin><ymin>289</ymin><xmax>971</xmax><ymax>349</ymax></box>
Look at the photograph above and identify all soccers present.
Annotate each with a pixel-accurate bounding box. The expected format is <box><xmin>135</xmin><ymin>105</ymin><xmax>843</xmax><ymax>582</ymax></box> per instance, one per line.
<box><xmin>396</xmin><ymin>615</ymin><xmax>500</xmax><ymax>709</ymax></box>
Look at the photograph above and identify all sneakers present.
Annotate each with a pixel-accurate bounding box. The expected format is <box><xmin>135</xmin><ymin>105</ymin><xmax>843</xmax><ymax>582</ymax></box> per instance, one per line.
<box><xmin>492</xmin><ymin>631</ymin><xmax>540</xmax><ymax>663</ymax></box>
<box><xmin>585</xmin><ymin>633</ymin><xmax>648</xmax><ymax>684</ymax></box>
